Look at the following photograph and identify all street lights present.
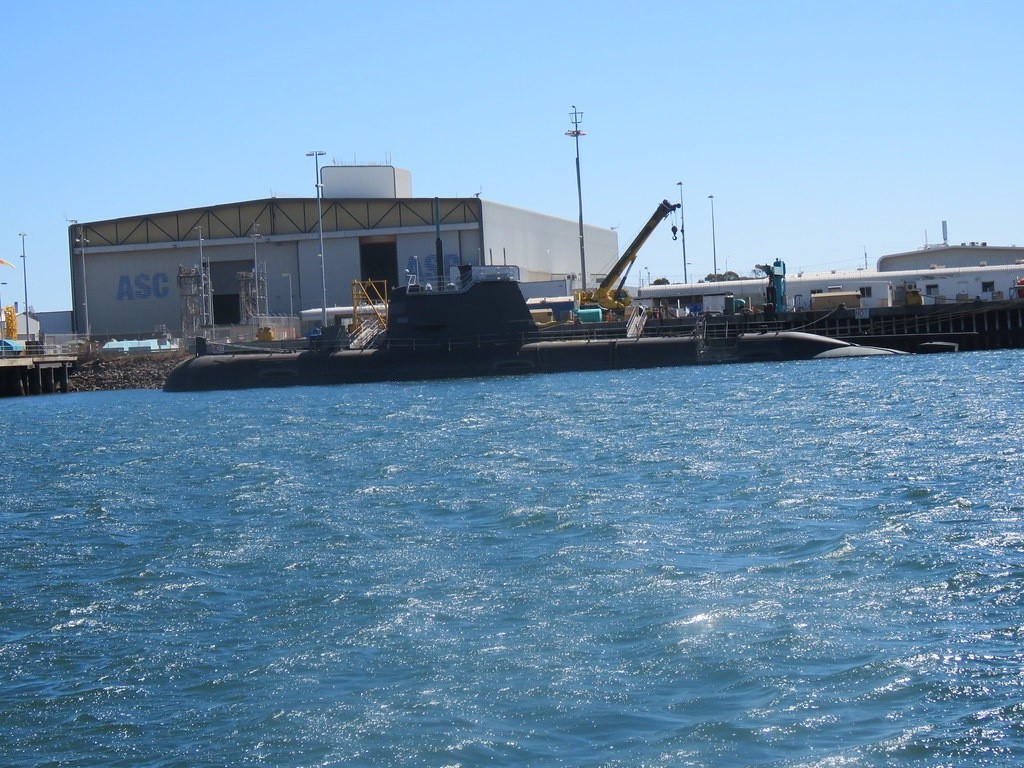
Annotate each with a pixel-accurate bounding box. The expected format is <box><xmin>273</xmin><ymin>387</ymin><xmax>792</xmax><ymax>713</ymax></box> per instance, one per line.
<box><xmin>564</xmin><ymin>104</ymin><xmax>587</xmax><ymax>289</ymax></box>
<box><xmin>305</xmin><ymin>149</ymin><xmax>328</xmax><ymax>328</ymax></box>
<box><xmin>19</xmin><ymin>232</ymin><xmax>31</xmax><ymax>341</ymax></box>
<box><xmin>707</xmin><ymin>194</ymin><xmax>717</xmax><ymax>274</ymax></box>
<box><xmin>676</xmin><ymin>180</ymin><xmax>688</xmax><ymax>284</ymax></box>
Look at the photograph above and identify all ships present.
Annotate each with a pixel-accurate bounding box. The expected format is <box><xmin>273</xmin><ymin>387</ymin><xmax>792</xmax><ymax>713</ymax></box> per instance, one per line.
<box><xmin>161</xmin><ymin>266</ymin><xmax>915</xmax><ymax>392</ymax></box>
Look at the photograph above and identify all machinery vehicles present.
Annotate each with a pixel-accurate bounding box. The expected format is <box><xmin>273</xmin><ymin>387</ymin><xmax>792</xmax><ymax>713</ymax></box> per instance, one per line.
<box><xmin>574</xmin><ymin>199</ymin><xmax>682</xmax><ymax>323</ymax></box>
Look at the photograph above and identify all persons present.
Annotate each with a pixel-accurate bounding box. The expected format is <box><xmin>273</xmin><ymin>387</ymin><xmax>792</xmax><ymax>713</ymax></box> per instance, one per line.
<box><xmin>256</xmin><ymin>326</ymin><xmax>275</xmax><ymax>342</ymax></box>
<box><xmin>111</xmin><ymin>336</ymin><xmax>118</xmax><ymax>342</ymax></box>
